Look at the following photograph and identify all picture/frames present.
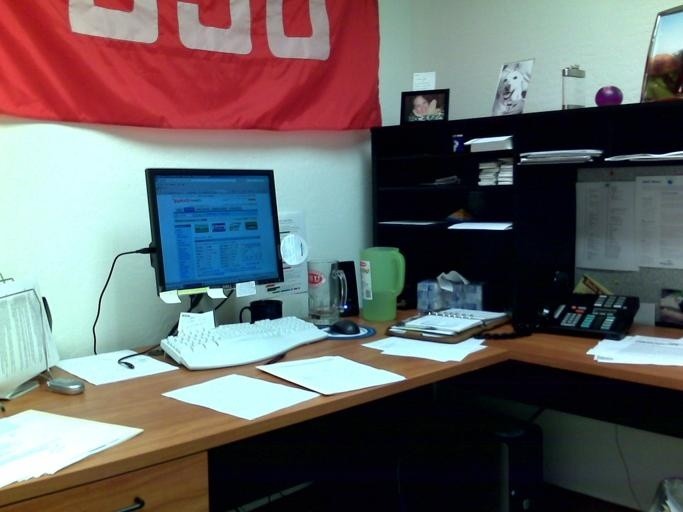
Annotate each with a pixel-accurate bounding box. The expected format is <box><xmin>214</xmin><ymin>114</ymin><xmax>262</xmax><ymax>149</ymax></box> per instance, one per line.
<box><xmin>397</xmin><ymin>88</ymin><xmax>451</xmax><ymax>124</ymax></box>
<box><xmin>639</xmin><ymin>3</ymin><xmax>683</xmax><ymax>103</ymax></box>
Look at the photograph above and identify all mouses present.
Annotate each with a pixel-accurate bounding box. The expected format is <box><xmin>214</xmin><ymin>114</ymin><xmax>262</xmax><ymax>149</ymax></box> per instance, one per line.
<box><xmin>330</xmin><ymin>320</ymin><xmax>360</xmax><ymax>335</ymax></box>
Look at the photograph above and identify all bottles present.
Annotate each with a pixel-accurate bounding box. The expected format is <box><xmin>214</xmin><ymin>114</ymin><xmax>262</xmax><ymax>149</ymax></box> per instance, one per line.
<box><xmin>562</xmin><ymin>64</ymin><xmax>587</xmax><ymax>109</ymax></box>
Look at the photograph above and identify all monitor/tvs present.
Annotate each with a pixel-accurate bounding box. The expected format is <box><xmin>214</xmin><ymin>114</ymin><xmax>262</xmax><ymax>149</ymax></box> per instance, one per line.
<box><xmin>145</xmin><ymin>168</ymin><xmax>285</xmax><ymax>299</ymax></box>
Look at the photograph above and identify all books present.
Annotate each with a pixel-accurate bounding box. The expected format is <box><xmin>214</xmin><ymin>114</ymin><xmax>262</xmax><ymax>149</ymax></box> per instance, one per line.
<box><xmin>518</xmin><ymin>149</ymin><xmax>682</xmax><ymax>163</ymax></box>
<box><xmin>431</xmin><ymin>156</ymin><xmax>512</xmax><ymax>187</ymax></box>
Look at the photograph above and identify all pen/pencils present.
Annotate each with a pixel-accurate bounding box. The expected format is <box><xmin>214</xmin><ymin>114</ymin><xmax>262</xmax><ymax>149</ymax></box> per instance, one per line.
<box><xmin>0</xmin><ymin>405</ymin><xmax>5</xmax><ymax>412</ymax></box>
<box><xmin>266</xmin><ymin>353</ymin><xmax>286</xmax><ymax>365</ymax></box>
<box><xmin>398</xmin><ymin>325</ymin><xmax>438</xmax><ymax>329</ymax></box>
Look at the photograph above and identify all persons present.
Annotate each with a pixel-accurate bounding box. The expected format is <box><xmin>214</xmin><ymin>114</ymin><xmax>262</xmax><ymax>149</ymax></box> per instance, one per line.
<box><xmin>408</xmin><ymin>94</ymin><xmax>443</xmax><ymax>121</ymax></box>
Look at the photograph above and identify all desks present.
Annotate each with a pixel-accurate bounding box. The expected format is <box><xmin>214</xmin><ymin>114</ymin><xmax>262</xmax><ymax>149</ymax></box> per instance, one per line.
<box><xmin>0</xmin><ymin>305</ymin><xmax>683</xmax><ymax>511</ymax></box>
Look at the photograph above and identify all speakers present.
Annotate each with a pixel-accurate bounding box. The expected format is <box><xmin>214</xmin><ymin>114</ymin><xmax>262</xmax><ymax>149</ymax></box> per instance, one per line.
<box><xmin>328</xmin><ymin>261</ymin><xmax>360</xmax><ymax>317</ymax></box>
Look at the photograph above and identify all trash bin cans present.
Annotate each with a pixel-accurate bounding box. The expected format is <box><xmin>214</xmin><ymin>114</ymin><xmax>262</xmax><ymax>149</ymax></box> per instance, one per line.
<box><xmin>649</xmin><ymin>477</ymin><xmax>683</xmax><ymax>511</ymax></box>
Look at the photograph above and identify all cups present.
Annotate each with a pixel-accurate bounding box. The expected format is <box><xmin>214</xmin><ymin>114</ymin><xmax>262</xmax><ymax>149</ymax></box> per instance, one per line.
<box><xmin>360</xmin><ymin>246</ymin><xmax>406</xmax><ymax>321</ymax></box>
<box><xmin>239</xmin><ymin>299</ymin><xmax>283</xmax><ymax>324</ymax></box>
<box><xmin>308</xmin><ymin>258</ymin><xmax>346</xmax><ymax>326</ymax></box>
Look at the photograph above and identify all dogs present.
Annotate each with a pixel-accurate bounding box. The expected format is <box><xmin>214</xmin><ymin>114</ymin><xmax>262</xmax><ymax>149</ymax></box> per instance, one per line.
<box><xmin>491</xmin><ymin>63</ymin><xmax>529</xmax><ymax>115</ymax></box>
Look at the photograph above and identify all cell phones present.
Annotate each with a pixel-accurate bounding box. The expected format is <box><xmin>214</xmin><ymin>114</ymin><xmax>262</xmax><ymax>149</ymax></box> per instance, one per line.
<box><xmin>46</xmin><ymin>377</ymin><xmax>85</xmax><ymax>395</ymax></box>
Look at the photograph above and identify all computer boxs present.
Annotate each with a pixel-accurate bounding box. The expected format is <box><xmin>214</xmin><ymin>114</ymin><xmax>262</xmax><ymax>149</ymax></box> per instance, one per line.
<box><xmin>395</xmin><ymin>403</ymin><xmax>543</xmax><ymax>512</ymax></box>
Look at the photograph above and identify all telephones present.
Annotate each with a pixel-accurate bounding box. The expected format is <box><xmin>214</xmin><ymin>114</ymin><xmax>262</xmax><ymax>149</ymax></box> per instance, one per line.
<box><xmin>511</xmin><ymin>265</ymin><xmax>641</xmax><ymax>341</ymax></box>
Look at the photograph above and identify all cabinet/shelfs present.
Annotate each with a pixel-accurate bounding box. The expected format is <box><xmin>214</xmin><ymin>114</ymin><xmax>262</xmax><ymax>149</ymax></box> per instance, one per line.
<box><xmin>369</xmin><ymin>107</ymin><xmax>578</xmax><ymax>311</ymax></box>
<box><xmin>0</xmin><ymin>451</ymin><xmax>209</xmax><ymax>512</ymax></box>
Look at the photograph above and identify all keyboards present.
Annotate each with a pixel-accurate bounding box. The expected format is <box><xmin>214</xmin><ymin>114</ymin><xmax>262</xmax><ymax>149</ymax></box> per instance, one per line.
<box><xmin>160</xmin><ymin>316</ymin><xmax>329</xmax><ymax>371</ymax></box>
